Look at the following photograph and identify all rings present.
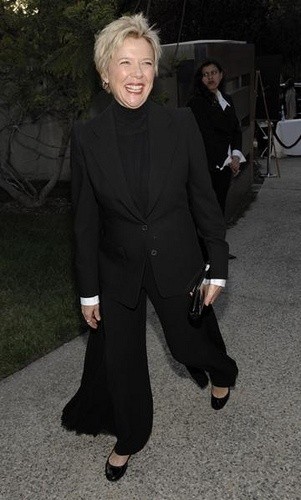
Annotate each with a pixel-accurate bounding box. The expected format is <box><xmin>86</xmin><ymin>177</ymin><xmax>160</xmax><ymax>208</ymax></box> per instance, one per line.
<box><xmin>85</xmin><ymin>319</ymin><xmax>91</xmax><ymax>323</ymax></box>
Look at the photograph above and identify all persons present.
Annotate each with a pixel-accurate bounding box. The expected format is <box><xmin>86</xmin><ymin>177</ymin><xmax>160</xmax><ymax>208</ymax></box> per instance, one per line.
<box><xmin>61</xmin><ymin>11</ymin><xmax>240</xmax><ymax>482</ymax></box>
<box><xmin>186</xmin><ymin>60</ymin><xmax>244</xmax><ymax>259</ymax></box>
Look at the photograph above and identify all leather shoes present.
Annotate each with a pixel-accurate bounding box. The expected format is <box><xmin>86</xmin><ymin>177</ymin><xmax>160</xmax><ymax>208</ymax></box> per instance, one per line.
<box><xmin>104</xmin><ymin>442</ymin><xmax>130</xmax><ymax>483</ymax></box>
<box><xmin>210</xmin><ymin>385</ymin><xmax>231</xmax><ymax>410</ymax></box>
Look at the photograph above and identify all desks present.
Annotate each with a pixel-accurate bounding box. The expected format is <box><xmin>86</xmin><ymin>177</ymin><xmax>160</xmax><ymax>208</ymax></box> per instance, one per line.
<box><xmin>271</xmin><ymin>119</ymin><xmax>301</xmax><ymax>159</ymax></box>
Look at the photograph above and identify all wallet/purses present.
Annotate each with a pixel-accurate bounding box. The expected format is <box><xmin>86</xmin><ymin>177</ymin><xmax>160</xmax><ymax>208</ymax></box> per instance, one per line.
<box><xmin>185</xmin><ymin>262</ymin><xmax>210</xmax><ymax>319</ymax></box>
